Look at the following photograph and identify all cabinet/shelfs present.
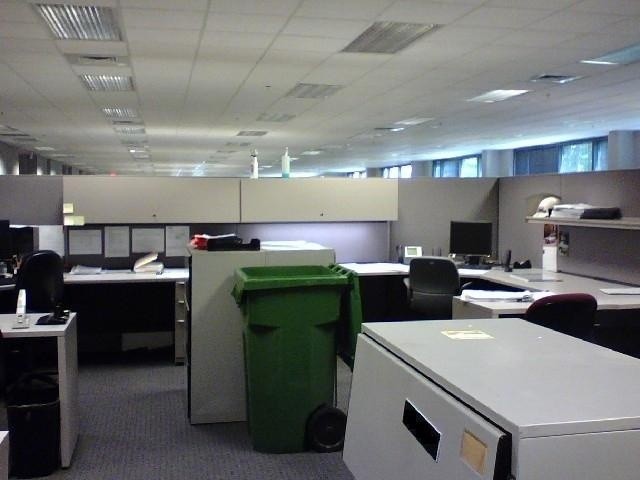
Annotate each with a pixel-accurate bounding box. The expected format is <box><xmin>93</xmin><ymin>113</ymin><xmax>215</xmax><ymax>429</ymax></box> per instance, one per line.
<box><xmin>183</xmin><ymin>240</ymin><xmax>341</xmax><ymax>428</ymax></box>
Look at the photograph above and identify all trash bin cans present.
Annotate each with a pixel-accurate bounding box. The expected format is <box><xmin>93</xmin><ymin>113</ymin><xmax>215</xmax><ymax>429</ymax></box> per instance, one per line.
<box><xmin>5</xmin><ymin>370</ymin><xmax>60</xmax><ymax>479</ymax></box>
<box><xmin>231</xmin><ymin>265</ymin><xmax>362</xmax><ymax>453</ymax></box>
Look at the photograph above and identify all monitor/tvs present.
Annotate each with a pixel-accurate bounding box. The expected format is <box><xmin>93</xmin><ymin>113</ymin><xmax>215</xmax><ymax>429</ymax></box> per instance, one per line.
<box><xmin>450</xmin><ymin>221</ymin><xmax>492</xmax><ymax>265</ymax></box>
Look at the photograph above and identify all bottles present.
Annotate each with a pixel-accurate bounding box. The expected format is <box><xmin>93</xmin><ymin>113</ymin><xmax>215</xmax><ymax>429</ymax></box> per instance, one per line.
<box><xmin>281</xmin><ymin>146</ymin><xmax>290</xmax><ymax>178</ymax></box>
<box><xmin>250</xmin><ymin>154</ymin><xmax>259</xmax><ymax>179</ymax></box>
<box><xmin>0</xmin><ymin>254</ymin><xmax>18</xmax><ymax>279</ymax></box>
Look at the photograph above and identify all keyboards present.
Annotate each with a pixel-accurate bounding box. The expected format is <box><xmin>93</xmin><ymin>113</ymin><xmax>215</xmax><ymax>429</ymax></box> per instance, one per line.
<box><xmin>456</xmin><ymin>264</ymin><xmax>491</xmax><ymax>270</ymax></box>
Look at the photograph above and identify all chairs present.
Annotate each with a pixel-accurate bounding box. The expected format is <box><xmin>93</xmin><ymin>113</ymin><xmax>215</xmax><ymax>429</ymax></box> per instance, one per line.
<box><xmin>403</xmin><ymin>257</ymin><xmax>475</xmax><ymax>320</ymax></box>
<box><xmin>526</xmin><ymin>293</ymin><xmax>601</xmax><ymax>344</ymax></box>
<box><xmin>12</xmin><ymin>249</ymin><xmax>67</xmax><ymax>311</ymax></box>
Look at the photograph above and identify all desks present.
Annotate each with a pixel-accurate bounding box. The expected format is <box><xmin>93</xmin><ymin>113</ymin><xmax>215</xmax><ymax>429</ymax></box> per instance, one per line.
<box><xmin>1</xmin><ymin>311</ymin><xmax>83</xmax><ymax>480</ymax></box>
<box><xmin>0</xmin><ymin>265</ymin><xmax>189</xmax><ymax>368</ymax></box>
<box><xmin>341</xmin><ymin>258</ymin><xmax>640</xmax><ymax>324</ymax></box>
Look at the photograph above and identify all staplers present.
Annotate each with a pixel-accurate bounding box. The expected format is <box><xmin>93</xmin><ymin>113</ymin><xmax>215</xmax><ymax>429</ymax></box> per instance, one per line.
<box><xmin>12</xmin><ymin>288</ymin><xmax>30</xmax><ymax>329</ymax></box>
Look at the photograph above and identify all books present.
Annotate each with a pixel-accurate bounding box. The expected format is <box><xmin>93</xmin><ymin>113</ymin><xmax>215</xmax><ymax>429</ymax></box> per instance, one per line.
<box><xmin>133</xmin><ymin>251</ymin><xmax>164</xmax><ymax>276</ymax></box>
